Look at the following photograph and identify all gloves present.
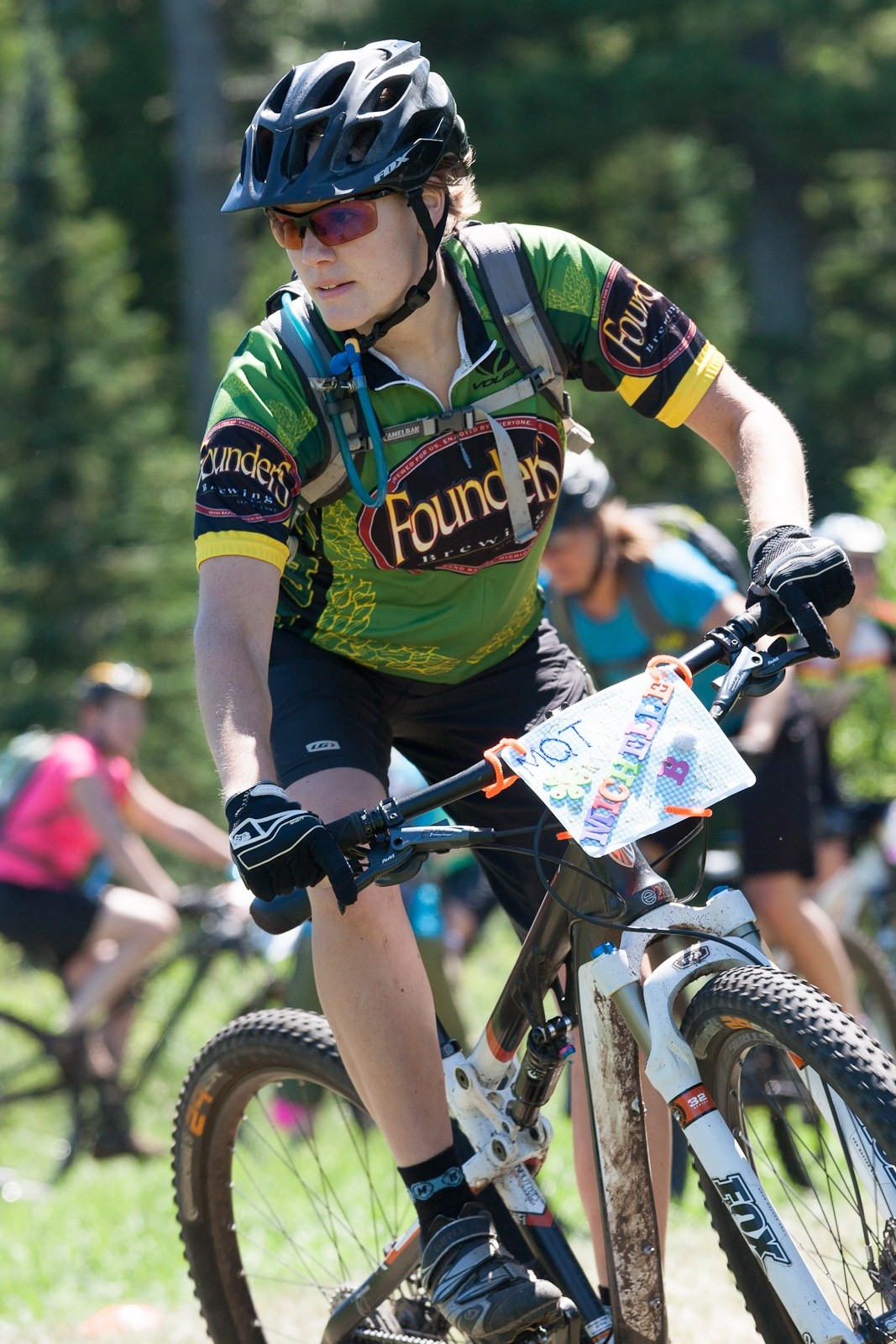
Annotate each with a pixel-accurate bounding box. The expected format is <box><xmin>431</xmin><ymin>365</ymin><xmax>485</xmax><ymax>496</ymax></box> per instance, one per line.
<box><xmin>220</xmin><ymin>778</ymin><xmax>361</xmax><ymax>910</ymax></box>
<box><xmin>740</xmin><ymin>528</ymin><xmax>862</xmax><ymax>666</ymax></box>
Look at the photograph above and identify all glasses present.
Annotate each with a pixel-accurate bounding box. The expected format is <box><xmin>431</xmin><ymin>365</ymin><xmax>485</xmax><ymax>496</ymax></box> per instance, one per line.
<box><xmin>264</xmin><ymin>187</ymin><xmax>400</xmax><ymax>251</ymax></box>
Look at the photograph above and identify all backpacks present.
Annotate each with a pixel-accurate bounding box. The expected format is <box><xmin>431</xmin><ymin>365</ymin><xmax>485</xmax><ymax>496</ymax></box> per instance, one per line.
<box><xmin>0</xmin><ymin>728</ymin><xmax>55</xmax><ymax>817</ymax></box>
<box><xmin>547</xmin><ymin>502</ymin><xmax>747</xmax><ymax>699</ymax></box>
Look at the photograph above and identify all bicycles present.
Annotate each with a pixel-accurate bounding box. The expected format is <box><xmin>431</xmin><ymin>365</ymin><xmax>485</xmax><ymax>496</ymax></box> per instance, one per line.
<box><xmin>166</xmin><ymin>596</ymin><xmax>896</xmax><ymax>1343</ymax></box>
<box><xmin>0</xmin><ymin>874</ymin><xmax>261</xmax><ymax>1200</ymax></box>
<box><xmin>231</xmin><ymin>737</ymin><xmax>896</xmax><ymax>1197</ymax></box>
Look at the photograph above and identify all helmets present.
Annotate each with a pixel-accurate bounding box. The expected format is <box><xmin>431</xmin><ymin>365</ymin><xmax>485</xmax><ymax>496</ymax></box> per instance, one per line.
<box><xmin>215</xmin><ymin>39</ymin><xmax>470</xmax><ymax>217</ymax></box>
<box><xmin>71</xmin><ymin>660</ymin><xmax>157</xmax><ymax>707</ymax></box>
<box><xmin>815</xmin><ymin>510</ymin><xmax>889</xmax><ymax>560</ymax></box>
<box><xmin>546</xmin><ymin>442</ymin><xmax>614</xmax><ymax>523</ymax></box>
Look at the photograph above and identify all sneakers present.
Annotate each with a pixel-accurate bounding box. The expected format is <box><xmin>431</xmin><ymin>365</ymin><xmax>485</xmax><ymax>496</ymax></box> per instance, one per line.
<box><xmin>411</xmin><ymin>1200</ymin><xmax>567</xmax><ymax>1343</ymax></box>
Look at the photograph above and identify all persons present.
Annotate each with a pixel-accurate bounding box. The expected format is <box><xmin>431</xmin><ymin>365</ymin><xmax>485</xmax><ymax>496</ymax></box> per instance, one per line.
<box><xmin>0</xmin><ymin>660</ymin><xmax>233</xmax><ymax>1157</ymax></box>
<box><xmin>195</xmin><ymin>39</ymin><xmax>856</xmax><ymax>1340</ymax></box>
<box><xmin>776</xmin><ymin>513</ymin><xmax>895</xmax><ymax>901</ymax></box>
<box><xmin>259</xmin><ymin>745</ymin><xmax>503</xmax><ymax>1143</ymax></box>
<box><xmin>533</xmin><ymin>445</ymin><xmax>854</xmax><ymax>1026</ymax></box>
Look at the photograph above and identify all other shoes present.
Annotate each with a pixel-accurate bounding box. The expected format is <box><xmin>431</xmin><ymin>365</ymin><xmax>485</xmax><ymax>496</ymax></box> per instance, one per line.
<box><xmin>93</xmin><ymin>1127</ymin><xmax>166</xmax><ymax>1165</ymax></box>
<box><xmin>54</xmin><ymin>1023</ymin><xmax>121</xmax><ymax>1087</ymax></box>
